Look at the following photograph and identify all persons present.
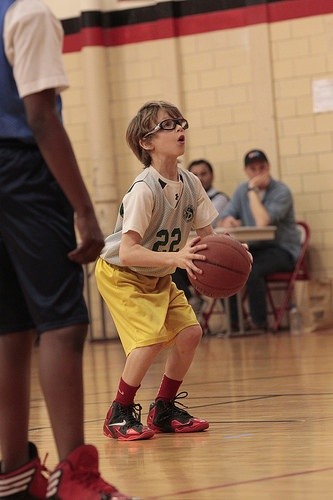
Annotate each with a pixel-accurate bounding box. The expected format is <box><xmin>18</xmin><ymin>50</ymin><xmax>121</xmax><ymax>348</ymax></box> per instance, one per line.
<box><xmin>95</xmin><ymin>101</ymin><xmax>254</xmax><ymax>440</ymax></box>
<box><xmin>218</xmin><ymin>149</ymin><xmax>302</xmax><ymax>336</ymax></box>
<box><xmin>0</xmin><ymin>1</ymin><xmax>130</xmax><ymax>500</ymax></box>
<box><xmin>171</xmin><ymin>159</ymin><xmax>231</xmax><ymax>323</ymax></box>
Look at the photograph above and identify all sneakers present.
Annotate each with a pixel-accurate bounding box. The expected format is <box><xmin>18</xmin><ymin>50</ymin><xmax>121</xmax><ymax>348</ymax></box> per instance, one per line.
<box><xmin>147</xmin><ymin>391</ymin><xmax>209</xmax><ymax>433</ymax></box>
<box><xmin>103</xmin><ymin>400</ymin><xmax>154</xmax><ymax>441</ymax></box>
<box><xmin>46</xmin><ymin>445</ymin><xmax>132</xmax><ymax>500</ymax></box>
<box><xmin>0</xmin><ymin>442</ymin><xmax>53</xmax><ymax>500</ymax></box>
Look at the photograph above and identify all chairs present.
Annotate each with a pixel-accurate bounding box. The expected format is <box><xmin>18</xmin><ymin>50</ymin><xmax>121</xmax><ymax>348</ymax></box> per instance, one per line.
<box><xmin>201</xmin><ymin>221</ymin><xmax>312</xmax><ymax>335</ymax></box>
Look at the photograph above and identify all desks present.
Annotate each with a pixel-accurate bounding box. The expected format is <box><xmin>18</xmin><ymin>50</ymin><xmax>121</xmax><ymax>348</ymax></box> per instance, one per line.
<box><xmin>79</xmin><ymin>225</ymin><xmax>278</xmax><ymax>345</ymax></box>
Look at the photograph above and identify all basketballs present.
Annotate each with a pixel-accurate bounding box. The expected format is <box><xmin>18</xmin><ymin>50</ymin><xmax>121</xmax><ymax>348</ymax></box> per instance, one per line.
<box><xmin>187</xmin><ymin>234</ymin><xmax>251</xmax><ymax>298</ymax></box>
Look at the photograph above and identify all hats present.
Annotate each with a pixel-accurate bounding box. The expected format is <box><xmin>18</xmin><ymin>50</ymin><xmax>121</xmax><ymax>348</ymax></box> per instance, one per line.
<box><xmin>245</xmin><ymin>150</ymin><xmax>266</xmax><ymax>165</ymax></box>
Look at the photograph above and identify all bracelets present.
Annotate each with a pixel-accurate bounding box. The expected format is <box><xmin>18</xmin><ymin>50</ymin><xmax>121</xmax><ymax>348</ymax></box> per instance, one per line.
<box><xmin>248</xmin><ymin>186</ymin><xmax>260</xmax><ymax>193</ymax></box>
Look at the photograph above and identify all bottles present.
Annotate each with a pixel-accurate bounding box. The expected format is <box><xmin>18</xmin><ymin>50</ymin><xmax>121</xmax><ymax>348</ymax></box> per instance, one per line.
<box><xmin>289</xmin><ymin>303</ymin><xmax>301</xmax><ymax>336</ymax></box>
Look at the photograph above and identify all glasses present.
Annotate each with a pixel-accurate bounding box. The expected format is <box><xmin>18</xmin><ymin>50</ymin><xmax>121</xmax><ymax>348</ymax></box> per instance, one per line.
<box><xmin>143</xmin><ymin>119</ymin><xmax>188</xmax><ymax>138</ymax></box>
